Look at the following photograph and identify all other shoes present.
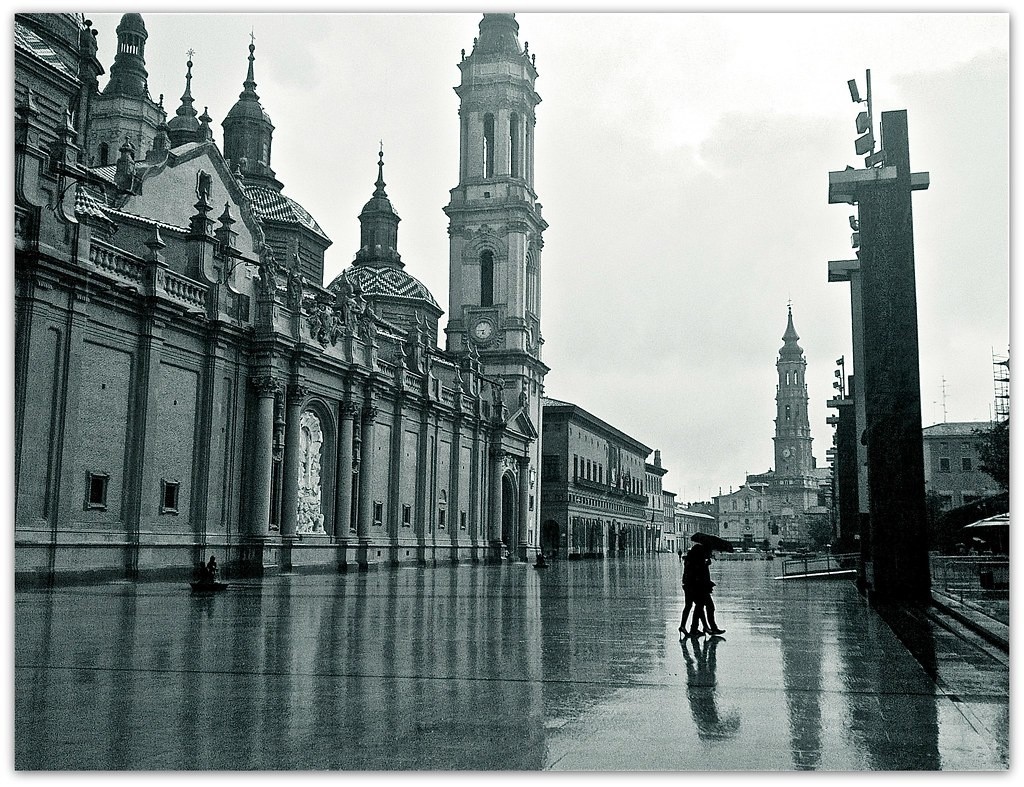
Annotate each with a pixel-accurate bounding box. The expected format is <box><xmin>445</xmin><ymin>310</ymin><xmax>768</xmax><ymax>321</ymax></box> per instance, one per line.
<box><xmin>712</xmin><ymin>636</ymin><xmax>726</xmax><ymax>644</ymax></box>
<box><xmin>688</xmin><ymin>625</ymin><xmax>705</xmax><ymax>636</ymax></box>
<box><xmin>691</xmin><ymin>634</ymin><xmax>704</xmax><ymax>640</ymax></box>
<box><xmin>712</xmin><ymin>625</ymin><xmax>726</xmax><ymax>634</ymax></box>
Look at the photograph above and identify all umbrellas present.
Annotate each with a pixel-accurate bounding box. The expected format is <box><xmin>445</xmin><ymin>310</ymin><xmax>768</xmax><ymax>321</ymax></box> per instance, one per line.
<box><xmin>962</xmin><ymin>512</ymin><xmax>1009</xmax><ymax>527</ymax></box>
<box><xmin>690</xmin><ymin>532</ymin><xmax>735</xmax><ymax>560</ymax></box>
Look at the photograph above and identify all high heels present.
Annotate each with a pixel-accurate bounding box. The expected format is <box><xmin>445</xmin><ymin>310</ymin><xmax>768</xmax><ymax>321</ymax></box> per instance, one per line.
<box><xmin>679</xmin><ymin>636</ymin><xmax>690</xmax><ymax>642</ymax></box>
<box><xmin>703</xmin><ymin>628</ymin><xmax>714</xmax><ymax>635</ymax></box>
<box><xmin>679</xmin><ymin>627</ymin><xmax>690</xmax><ymax>638</ymax></box>
<box><xmin>704</xmin><ymin>636</ymin><xmax>717</xmax><ymax>645</ymax></box>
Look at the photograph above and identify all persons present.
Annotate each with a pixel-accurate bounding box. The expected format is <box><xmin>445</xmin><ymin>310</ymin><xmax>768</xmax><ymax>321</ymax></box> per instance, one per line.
<box><xmin>193</xmin><ymin>555</ymin><xmax>217</xmax><ymax>584</ymax></box>
<box><xmin>258</xmin><ymin>246</ymin><xmax>359</xmax><ymax>349</ymax></box>
<box><xmin>536</xmin><ymin>552</ymin><xmax>547</xmax><ymax>566</ymax></box>
<box><xmin>678</xmin><ymin>543</ymin><xmax>727</xmax><ymax>637</ymax></box>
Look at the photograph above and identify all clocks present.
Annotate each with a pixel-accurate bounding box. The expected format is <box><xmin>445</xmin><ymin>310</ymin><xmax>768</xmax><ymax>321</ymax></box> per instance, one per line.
<box><xmin>782</xmin><ymin>449</ymin><xmax>791</xmax><ymax>458</ymax></box>
<box><xmin>474</xmin><ymin>319</ymin><xmax>493</xmax><ymax>340</ymax></box>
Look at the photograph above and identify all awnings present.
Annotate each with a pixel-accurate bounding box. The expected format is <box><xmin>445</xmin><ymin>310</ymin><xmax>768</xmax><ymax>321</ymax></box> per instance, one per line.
<box><xmin>938</xmin><ymin>489</ymin><xmax>955</xmax><ymax>496</ymax></box>
<box><xmin>962</xmin><ymin>489</ymin><xmax>1006</xmax><ymax>497</ymax></box>
<box><xmin>675</xmin><ymin>509</ymin><xmax>716</xmax><ymax>521</ymax></box>
